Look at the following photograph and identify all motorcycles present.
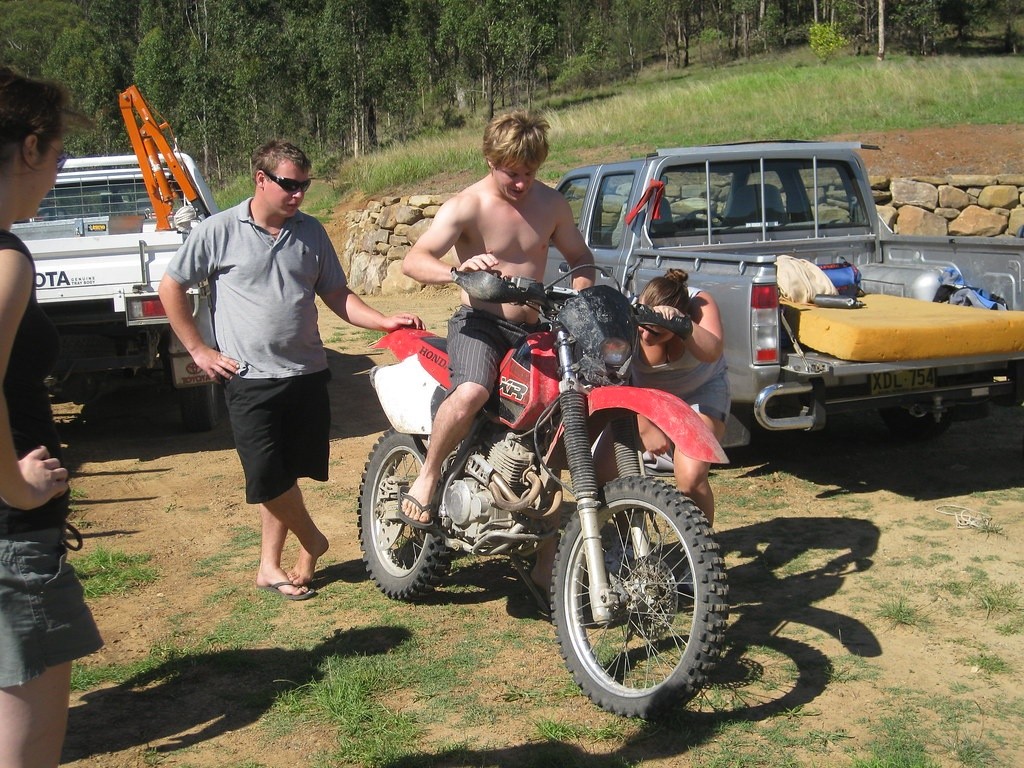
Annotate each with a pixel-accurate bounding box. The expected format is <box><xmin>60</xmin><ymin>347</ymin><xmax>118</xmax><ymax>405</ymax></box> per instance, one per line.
<box><xmin>356</xmin><ymin>263</ymin><xmax>729</xmax><ymax>724</ymax></box>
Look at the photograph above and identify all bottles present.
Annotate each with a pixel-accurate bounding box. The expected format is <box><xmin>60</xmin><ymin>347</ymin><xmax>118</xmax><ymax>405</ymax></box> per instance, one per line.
<box><xmin>815</xmin><ymin>294</ymin><xmax>866</xmax><ymax>308</ymax></box>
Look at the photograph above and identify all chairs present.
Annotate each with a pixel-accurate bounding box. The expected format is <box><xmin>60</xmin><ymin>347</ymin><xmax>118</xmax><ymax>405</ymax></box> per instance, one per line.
<box><xmin>610</xmin><ymin>196</ymin><xmax>676</xmax><ymax>248</ymax></box>
<box><xmin>717</xmin><ymin>183</ymin><xmax>787</xmax><ymax>227</ymax></box>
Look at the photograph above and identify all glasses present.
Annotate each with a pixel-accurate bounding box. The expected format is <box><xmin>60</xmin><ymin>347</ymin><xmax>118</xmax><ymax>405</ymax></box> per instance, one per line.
<box><xmin>263</xmin><ymin>170</ymin><xmax>311</xmax><ymax>192</ymax></box>
<box><xmin>48</xmin><ymin>141</ymin><xmax>68</xmax><ymax>172</ymax></box>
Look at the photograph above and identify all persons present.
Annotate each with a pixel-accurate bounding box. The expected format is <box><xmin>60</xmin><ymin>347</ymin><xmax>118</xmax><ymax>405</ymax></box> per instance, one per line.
<box><xmin>631</xmin><ymin>268</ymin><xmax>729</xmax><ymax>607</ymax></box>
<box><xmin>1</xmin><ymin>63</ymin><xmax>101</xmax><ymax>767</ymax></box>
<box><xmin>164</xmin><ymin>142</ymin><xmax>427</xmax><ymax>603</ymax></box>
<box><xmin>401</xmin><ymin>112</ymin><xmax>595</xmax><ymax>529</ymax></box>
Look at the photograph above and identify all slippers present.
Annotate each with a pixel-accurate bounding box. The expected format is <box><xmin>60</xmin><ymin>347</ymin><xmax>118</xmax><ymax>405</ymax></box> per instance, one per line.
<box><xmin>258</xmin><ymin>575</ymin><xmax>315</xmax><ymax>601</ymax></box>
<box><xmin>398</xmin><ymin>485</ymin><xmax>434</xmax><ymax>530</ymax></box>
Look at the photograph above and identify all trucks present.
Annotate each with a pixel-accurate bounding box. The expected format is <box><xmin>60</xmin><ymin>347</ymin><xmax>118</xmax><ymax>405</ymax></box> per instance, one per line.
<box><xmin>7</xmin><ymin>152</ymin><xmax>222</xmax><ymax>433</ymax></box>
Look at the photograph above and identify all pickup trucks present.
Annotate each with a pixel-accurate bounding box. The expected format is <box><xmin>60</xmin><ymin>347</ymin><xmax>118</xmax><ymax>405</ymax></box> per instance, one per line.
<box><xmin>527</xmin><ymin>142</ymin><xmax>1024</xmax><ymax>469</ymax></box>
